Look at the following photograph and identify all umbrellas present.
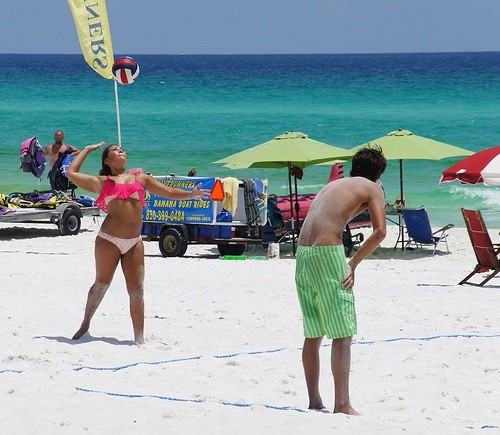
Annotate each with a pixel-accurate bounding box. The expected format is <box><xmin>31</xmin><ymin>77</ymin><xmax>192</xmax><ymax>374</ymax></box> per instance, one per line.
<box><xmin>210</xmin><ymin>128</ymin><xmax>500</xmax><ymax>257</ymax></box>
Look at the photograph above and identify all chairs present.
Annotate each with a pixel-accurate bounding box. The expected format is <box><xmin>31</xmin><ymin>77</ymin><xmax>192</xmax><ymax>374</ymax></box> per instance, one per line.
<box><xmin>458</xmin><ymin>207</ymin><xmax>500</xmax><ymax>288</ymax></box>
<box><xmin>262</xmin><ymin>194</ymin><xmax>300</xmax><ymax>249</ymax></box>
<box><xmin>399</xmin><ymin>207</ymin><xmax>454</xmax><ymax>256</ymax></box>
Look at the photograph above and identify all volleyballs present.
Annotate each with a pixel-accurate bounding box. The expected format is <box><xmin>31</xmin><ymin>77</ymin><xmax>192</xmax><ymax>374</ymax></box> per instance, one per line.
<box><xmin>111</xmin><ymin>56</ymin><xmax>140</xmax><ymax>84</ymax></box>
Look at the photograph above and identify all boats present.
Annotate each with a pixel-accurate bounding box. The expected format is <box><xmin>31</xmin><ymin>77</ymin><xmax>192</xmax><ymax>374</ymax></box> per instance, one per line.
<box><xmin>269</xmin><ymin>190</ymin><xmax>318</xmax><ymax>221</ymax></box>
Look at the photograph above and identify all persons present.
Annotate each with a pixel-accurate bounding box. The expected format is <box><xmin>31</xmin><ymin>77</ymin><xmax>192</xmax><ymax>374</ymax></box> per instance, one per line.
<box><xmin>41</xmin><ymin>131</ymin><xmax>80</xmax><ymax>193</ymax></box>
<box><xmin>68</xmin><ymin>141</ymin><xmax>210</xmax><ymax>347</ymax></box>
<box><xmin>296</xmin><ymin>145</ymin><xmax>389</xmax><ymax>415</ymax></box>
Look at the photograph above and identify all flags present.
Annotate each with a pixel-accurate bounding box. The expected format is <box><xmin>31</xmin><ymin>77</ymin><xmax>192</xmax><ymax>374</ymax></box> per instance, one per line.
<box><xmin>68</xmin><ymin>0</ymin><xmax>114</xmax><ymax>79</ymax></box>
